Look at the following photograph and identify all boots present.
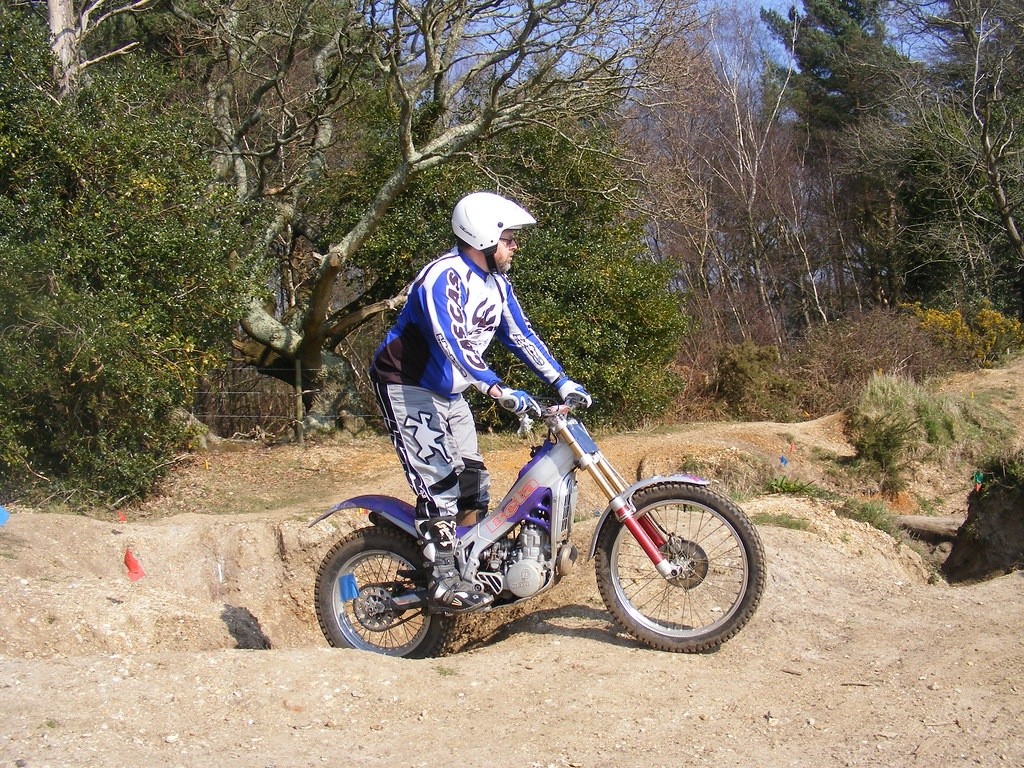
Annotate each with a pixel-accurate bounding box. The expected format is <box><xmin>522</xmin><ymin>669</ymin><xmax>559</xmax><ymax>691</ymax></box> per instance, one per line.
<box><xmin>413</xmin><ymin>516</ymin><xmax>494</xmax><ymax>612</ymax></box>
<box><xmin>456</xmin><ymin>506</ymin><xmax>487</xmax><ymax>526</ymax></box>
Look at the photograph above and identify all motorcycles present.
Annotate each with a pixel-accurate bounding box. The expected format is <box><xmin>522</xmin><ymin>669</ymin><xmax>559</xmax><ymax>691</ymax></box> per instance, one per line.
<box><xmin>305</xmin><ymin>393</ymin><xmax>768</xmax><ymax>662</ymax></box>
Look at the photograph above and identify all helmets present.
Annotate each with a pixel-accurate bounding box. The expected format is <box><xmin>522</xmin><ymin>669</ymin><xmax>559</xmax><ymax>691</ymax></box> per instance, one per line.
<box><xmin>452</xmin><ymin>193</ymin><xmax>536</xmax><ymax>274</ymax></box>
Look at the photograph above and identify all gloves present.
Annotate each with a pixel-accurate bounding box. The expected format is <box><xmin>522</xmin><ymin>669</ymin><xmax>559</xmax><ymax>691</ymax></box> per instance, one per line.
<box><xmin>491</xmin><ymin>382</ymin><xmax>541</xmax><ymax>417</ymax></box>
<box><xmin>555</xmin><ymin>377</ymin><xmax>592</xmax><ymax>408</ymax></box>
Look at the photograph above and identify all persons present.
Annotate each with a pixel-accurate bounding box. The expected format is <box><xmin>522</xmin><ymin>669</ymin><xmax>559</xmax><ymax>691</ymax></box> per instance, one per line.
<box><xmin>369</xmin><ymin>192</ymin><xmax>592</xmax><ymax>611</ymax></box>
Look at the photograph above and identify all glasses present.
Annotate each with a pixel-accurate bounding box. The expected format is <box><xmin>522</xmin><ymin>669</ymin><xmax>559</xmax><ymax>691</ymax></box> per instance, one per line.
<box><xmin>500</xmin><ymin>237</ymin><xmax>512</xmax><ymax>244</ymax></box>
